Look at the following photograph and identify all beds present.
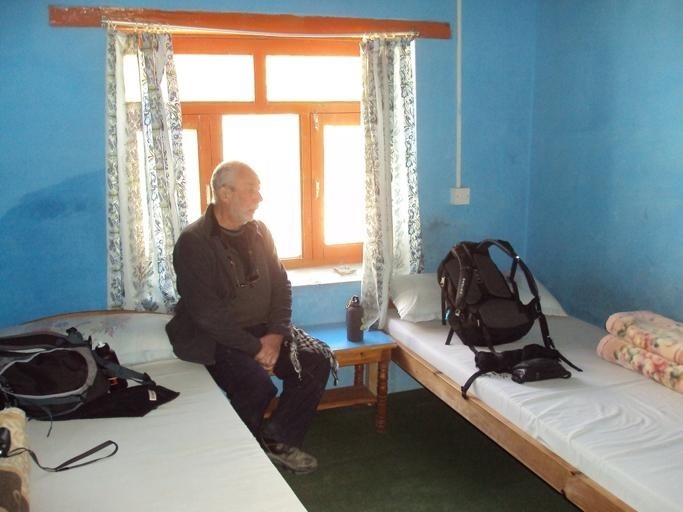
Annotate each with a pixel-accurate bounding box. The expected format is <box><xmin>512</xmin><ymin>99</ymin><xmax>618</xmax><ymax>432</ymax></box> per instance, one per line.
<box><xmin>386</xmin><ymin>264</ymin><xmax>683</xmax><ymax>512</ymax></box>
<box><xmin>2</xmin><ymin>309</ymin><xmax>312</xmax><ymax>512</ymax></box>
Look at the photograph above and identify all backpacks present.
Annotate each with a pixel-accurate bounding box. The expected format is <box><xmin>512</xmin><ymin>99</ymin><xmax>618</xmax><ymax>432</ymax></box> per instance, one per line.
<box><xmin>438</xmin><ymin>240</ymin><xmax>541</xmax><ymax>346</ymax></box>
<box><xmin>0</xmin><ymin>328</ymin><xmax>180</xmax><ymax>420</ymax></box>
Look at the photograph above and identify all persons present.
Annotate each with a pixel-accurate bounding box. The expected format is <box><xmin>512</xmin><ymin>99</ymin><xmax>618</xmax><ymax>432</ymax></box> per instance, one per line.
<box><xmin>165</xmin><ymin>160</ymin><xmax>332</xmax><ymax>477</ymax></box>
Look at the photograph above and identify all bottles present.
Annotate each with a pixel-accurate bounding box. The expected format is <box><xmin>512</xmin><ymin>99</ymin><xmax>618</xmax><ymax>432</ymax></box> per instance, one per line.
<box><xmin>95</xmin><ymin>340</ymin><xmax>127</xmax><ymax>391</ymax></box>
<box><xmin>346</xmin><ymin>295</ymin><xmax>364</xmax><ymax>341</ymax></box>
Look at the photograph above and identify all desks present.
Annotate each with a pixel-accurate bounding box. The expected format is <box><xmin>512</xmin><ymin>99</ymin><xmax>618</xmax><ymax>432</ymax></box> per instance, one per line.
<box><xmin>292</xmin><ymin>323</ymin><xmax>397</xmax><ymax>439</ymax></box>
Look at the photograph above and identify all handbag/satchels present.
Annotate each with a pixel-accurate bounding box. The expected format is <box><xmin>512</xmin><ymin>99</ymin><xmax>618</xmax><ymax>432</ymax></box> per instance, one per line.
<box><xmin>512</xmin><ymin>357</ymin><xmax>571</xmax><ymax>383</ymax></box>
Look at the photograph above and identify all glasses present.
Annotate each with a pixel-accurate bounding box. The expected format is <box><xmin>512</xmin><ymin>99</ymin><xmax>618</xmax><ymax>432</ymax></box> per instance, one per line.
<box><xmin>230</xmin><ymin>250</ymin><xmax>261</xmax><ymax>288</ymax></box>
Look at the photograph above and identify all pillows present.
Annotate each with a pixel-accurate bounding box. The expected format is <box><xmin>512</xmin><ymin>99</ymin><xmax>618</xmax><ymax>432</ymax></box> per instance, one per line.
<box><xmin>9</xmin><ymin>313</ymin><xmax>192</xmax><ymax>371</ymax></box>
<box><xmin>390</xmin><ymin>270</ymin><xmax>567</xmax><ymax>322</ymax></box>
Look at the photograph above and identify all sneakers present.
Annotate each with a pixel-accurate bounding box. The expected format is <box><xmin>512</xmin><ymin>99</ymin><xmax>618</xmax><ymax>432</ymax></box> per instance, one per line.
<box><xmin>263</xmin><ymin>443</ymin><xmax>318</xmax><ymax>475</ymax></box>
